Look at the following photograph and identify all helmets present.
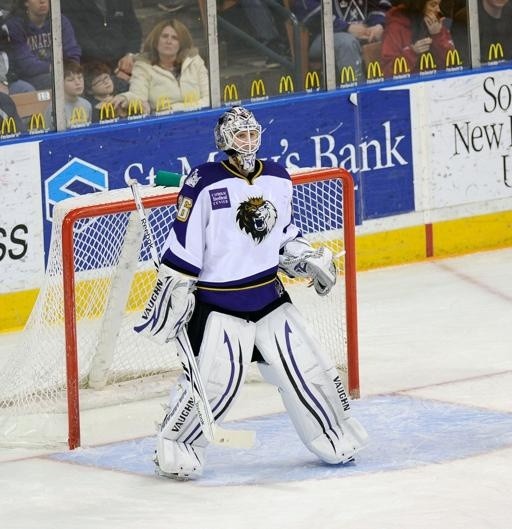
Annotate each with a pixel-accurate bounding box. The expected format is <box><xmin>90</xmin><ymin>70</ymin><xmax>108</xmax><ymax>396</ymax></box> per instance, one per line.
<box><xmin>214</xmin><ymin>106</ymin><xmax>262</xmax><ymax>152</ymax></box>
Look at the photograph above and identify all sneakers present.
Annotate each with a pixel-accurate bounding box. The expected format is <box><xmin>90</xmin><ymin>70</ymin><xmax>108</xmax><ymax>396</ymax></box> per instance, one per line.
<box><xmin>311</xmin><ymin>422</ymin><xmax>364</xmax><ymax>462</ymax></box>
<box><xmin>154</xmin><ymin>434</ymin><xmax>203</xmax><ymax>478</ymax></box>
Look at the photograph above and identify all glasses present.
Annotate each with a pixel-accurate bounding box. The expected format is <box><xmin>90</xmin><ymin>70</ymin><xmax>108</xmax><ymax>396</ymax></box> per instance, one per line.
<box><xmin>88</xmin><ymin>74</ymin><xmax>111</xmax><ymax>89</ymax></box>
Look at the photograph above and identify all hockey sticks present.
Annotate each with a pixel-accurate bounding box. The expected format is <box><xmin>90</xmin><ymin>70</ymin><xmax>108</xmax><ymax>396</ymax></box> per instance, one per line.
<box><xmin>127</xmin><ymin>179</ymin><xmax>258</xmax><ymax>449</ymax></box>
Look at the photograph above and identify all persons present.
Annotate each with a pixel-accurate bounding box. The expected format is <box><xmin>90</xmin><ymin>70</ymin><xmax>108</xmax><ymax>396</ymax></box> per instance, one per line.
<box><xmin>135</xmin><ymin>103</ymin><xmax>363</xmax><ymax>479</ymax></box>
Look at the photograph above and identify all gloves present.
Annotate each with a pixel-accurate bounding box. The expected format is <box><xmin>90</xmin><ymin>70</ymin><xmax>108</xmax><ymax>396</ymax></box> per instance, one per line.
<box><xmin>304</xmin><ymin>258</ymin><xmax>335</xmax><ymax>296</ymax></box>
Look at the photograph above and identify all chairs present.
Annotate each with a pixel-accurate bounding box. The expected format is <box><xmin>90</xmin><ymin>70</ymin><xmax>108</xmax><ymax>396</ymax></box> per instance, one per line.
<box><xmin>6</xmin><ymin>89</ymin><xmax>56</xmax><ymax>133</ymax></box>
